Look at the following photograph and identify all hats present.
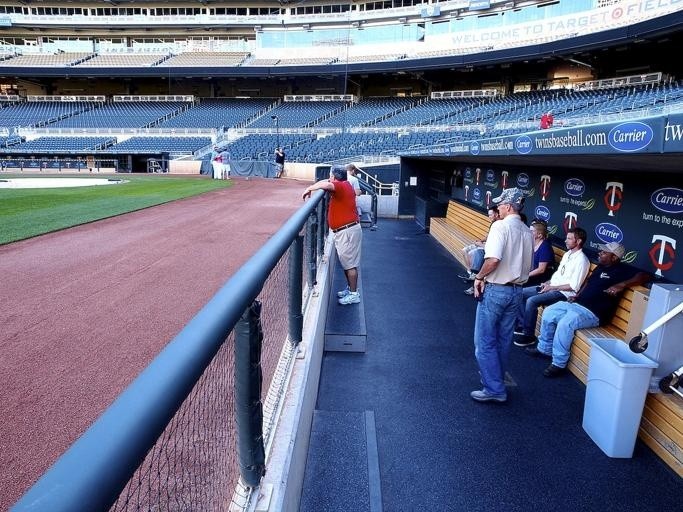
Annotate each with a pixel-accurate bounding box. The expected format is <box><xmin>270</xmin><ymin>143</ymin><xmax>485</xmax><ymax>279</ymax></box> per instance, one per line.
<box><xmin>597</xmin><ymin>242</ymin><xmax>625</xmax><ymax>260</ymax></box>
<box><xmin>492</xmin><ymin>188</ymin><xmax>526</xmax><ymax>205</ymax></box>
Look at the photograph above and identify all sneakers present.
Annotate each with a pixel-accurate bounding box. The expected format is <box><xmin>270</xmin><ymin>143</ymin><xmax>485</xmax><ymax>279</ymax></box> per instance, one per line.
<box><xmin>513</xmin><ymin>336</ymin><xmax>536</xmax><ymax>346</ymax></box>
<box><xmin>337</xmin><ymin>285</ymin><xmax>361</xmax><ymax>305</ymax></box>
<box><xmin>455</xmin><ymin>271</ymin><xmax>478</xmax><ymax>283</ymax></box>
<box><xmin>513</xmin><ymin>330</ymin><xmax>525</xmax><ymax>336</ymax></box>
<box><xmin>471</xmin><ymin>388</ymin><xmax>508</xmax><ymax>402</ymax></box>
<box><xmin>463</xmin><ymin>286</ymin><xmax>474</xmax><ymax>295</ymax></box>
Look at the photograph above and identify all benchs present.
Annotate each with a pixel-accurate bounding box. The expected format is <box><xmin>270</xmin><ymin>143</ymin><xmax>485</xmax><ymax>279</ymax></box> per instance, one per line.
<box><xmin>427</xmin><ymin>200</ymin><xmax>681</xmax><ymax>482</ymax></box>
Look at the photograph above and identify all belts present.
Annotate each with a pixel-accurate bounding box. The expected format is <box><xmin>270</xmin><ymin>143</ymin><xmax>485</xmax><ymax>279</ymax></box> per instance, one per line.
<box><xmin>332</xmin><ymin>221</ymin><xmax>359</xmax><ymax>233</ymax></box>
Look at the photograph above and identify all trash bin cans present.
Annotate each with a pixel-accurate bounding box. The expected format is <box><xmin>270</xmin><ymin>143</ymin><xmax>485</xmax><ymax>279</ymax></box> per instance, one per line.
<box><xmin>582</xmin><ymin>338</ymin><xmax>659</xmax><ymax>458</ymax></box>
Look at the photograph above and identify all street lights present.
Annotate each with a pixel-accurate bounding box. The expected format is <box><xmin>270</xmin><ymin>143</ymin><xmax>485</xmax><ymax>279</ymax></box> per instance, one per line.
<box><xmin>270</xmin><ymin>115</ymin><xmax>280</xmax><ymax>148</ymax></box>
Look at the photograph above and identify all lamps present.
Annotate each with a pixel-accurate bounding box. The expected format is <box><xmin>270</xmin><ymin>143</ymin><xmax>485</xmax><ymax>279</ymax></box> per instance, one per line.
<box><xmin>252</xmin><ymin>1</ymin><xmax>515</xmax><ymax>33</ymax></box>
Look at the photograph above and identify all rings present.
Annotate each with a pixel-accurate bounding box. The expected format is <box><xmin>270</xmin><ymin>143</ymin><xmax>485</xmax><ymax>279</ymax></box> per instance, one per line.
<box><xmin>611</xmin><ymin>290</ymin><xmax>614</xmax><ymax>292</ymax></box>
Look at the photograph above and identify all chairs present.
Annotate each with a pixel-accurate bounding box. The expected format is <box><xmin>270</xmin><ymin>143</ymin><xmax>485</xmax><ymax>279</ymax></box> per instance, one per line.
<box><xmin>0</xmin><ymin>36</ymin><xmax>567</xmax><ymax>68</ymax></box>
<box><xmin>2</xmin><ymin>81</ymin><xmax>683</xmax><ymax>168</ymax></box>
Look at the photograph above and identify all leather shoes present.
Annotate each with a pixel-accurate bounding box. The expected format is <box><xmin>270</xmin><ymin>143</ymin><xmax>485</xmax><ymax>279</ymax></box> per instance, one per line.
<box><xmin>543</xmin><ymin>364</ymin><xmax>564</xmax><ymax>376</ymax></box>
<box><xmin>524</xmin><ymin>346</ymin><xmax>551</xmax><ymax>360</ymax></box>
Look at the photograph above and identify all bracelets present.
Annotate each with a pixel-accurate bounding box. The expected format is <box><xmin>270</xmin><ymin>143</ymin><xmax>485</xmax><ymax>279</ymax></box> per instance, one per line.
<box><xmin>474</xmin><ymin>276</ymin><xmax>485</xmax><ymax>281</ymax></box>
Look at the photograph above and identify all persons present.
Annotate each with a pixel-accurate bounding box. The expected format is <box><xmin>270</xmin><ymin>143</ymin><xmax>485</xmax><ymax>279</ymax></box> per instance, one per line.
<box><xmin>463</xmin><ymin>217</ymin><xmax>548</xmax><ymax>296</ymax></box>
<box><xmin>345</xmin><ymin>163</ymin><xmax>362</xmax><ymax>221</ymax></box>
<box><xmin>509</xmin><ymin>227</ymin><xmax>590</xmax><ymax>347</ymax></box>
<box><xmin>518</xmin><ymin>224</ymin><xmax>558</xmax><ymax>287</ymax></box>
<box><xmin>469</xmin><ymin>186</ymin><xmax>531</xmax><ymax>403</ymax></box>
<box><xmin>300</xmin><ymin>166</ymin><xmax>363</xmax><ymax>305</ymax></box>
<box><xmin>273</xmin><ymin>147</ymin><xmax>284</xmax><ymax>179</ymax></box>
<box><xmin>209</xmin><ymin>146</ymin><xmax>233</xmax><ymax>181</ymax></box>
<box><xmin>453</xmin><ymin>205</ymin><xmax>498</xmax><ymax>283</ymax></box>
<box><xmin>537</xmin><ymin>110</ymin><xmax>554</xmax><ymax>131</ymax></box>
<box><xmin>523</xmin><ymin>240</ymin><xmax>653</xmax><ymax>378</ymax></box>
